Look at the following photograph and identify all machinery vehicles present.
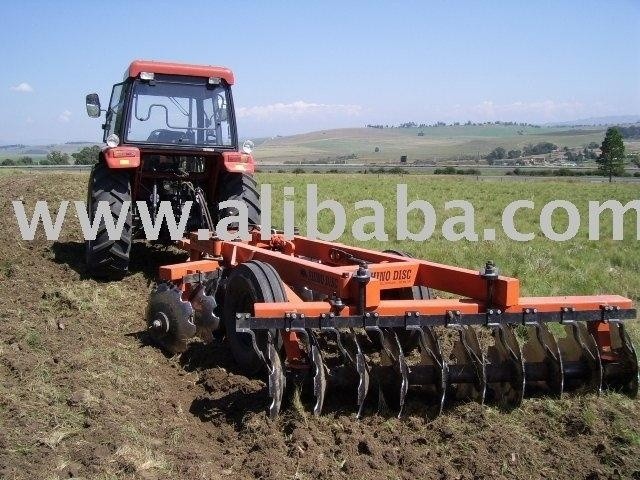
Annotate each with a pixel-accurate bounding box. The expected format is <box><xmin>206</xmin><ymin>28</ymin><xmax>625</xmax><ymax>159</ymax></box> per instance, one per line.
<box><xmin>86</xmin><ymin>60</ymin><xmax>638</xmax><ymax>418</ymax></box>
